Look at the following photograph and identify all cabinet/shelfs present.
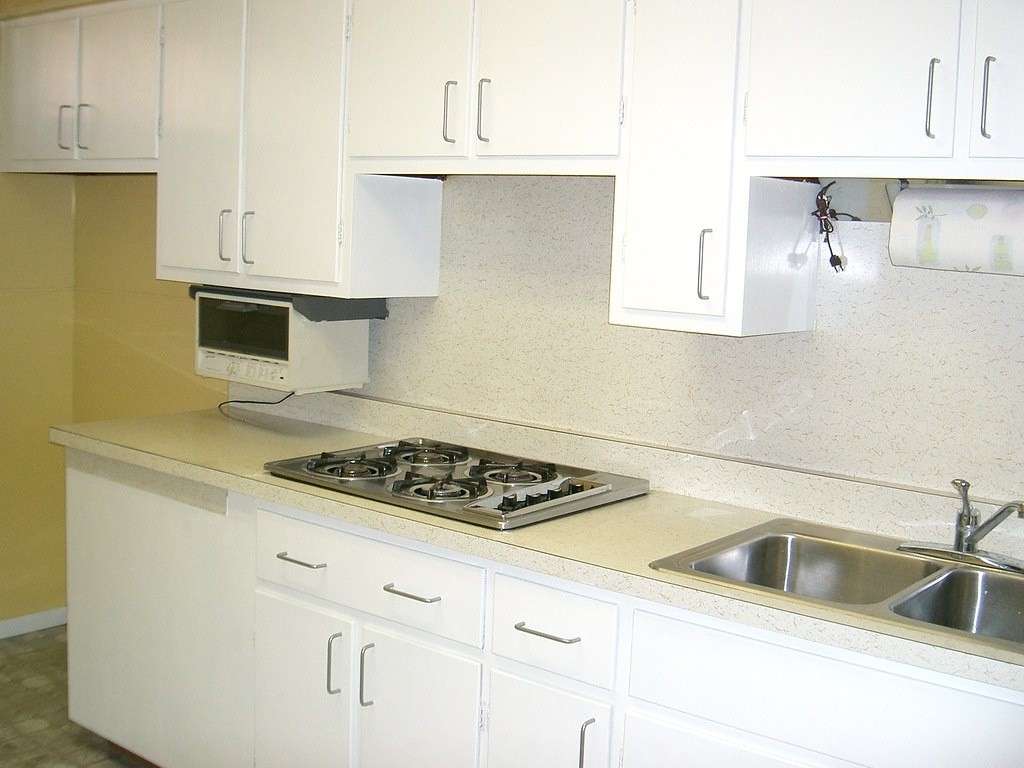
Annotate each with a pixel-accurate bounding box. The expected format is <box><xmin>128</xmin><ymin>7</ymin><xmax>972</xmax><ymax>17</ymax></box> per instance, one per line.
<box><xmin>0</xmin><ymin>0</ymin><xmax>159</xmax><ymax>173</ymax></box>
<box><xmin>487</xmin><ymin>561</ymin><xmax>618</xmax><ymax>768</ymax></box>
<box><xmin>745</xmin><ymin>0</ymin><xmax>1024</xmax><ymax>181</ymax></box>
<box><xmin>155</xmin><ymin>0</ymin><xmax>442</xmax><ymax>298</ymax></box>
<box><xmin>620</xmin><ymin>604</ymin><xmax>1024</xmax><ymax>768</ymax></box>
<box><xmin>254</xmin><ymin>497</ymin><xmax>485</xmax><ymax>768</ymax></box>
<box><xmin>347</xmin><ymin>1</ymin><xmax>621</xmax><ymax>177</ymax></box>
<box><xmin>608</xmin><ymin>1</ymin><xmax>817</xmax><ymax>338</ymax></box>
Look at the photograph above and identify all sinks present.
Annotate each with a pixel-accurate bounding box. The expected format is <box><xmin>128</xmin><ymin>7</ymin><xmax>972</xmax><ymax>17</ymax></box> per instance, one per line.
<box><xmin>893</xmin><ymin>568</ymin><xmax>1024</xmax><ymax>645</ymax></box>
<box><xmin>686</xmin><ymin>536</ymin><xmax>944</xmax><ymax>604</ymax></box>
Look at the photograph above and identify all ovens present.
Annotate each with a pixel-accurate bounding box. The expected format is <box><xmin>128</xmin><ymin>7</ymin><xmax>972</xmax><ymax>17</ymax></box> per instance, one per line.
<box><xmin>194</xmin><ymin>291</ymin><xmax>371</xmax><ymax>395</ymax></box>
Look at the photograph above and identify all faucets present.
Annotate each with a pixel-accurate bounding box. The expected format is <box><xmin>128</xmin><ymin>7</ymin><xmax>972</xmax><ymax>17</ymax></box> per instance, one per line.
<box><xmin>897</xmin><ymin>479</ymin><xmax>1024</xmax><ymax>574</ymax></box>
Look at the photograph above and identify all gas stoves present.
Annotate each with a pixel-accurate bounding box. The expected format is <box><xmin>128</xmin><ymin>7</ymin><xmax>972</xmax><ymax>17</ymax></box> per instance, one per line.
<box><xmin>263</xmin><ymin>437</ymin><xmax>650</xmax><ymax>531</ymax></box>
